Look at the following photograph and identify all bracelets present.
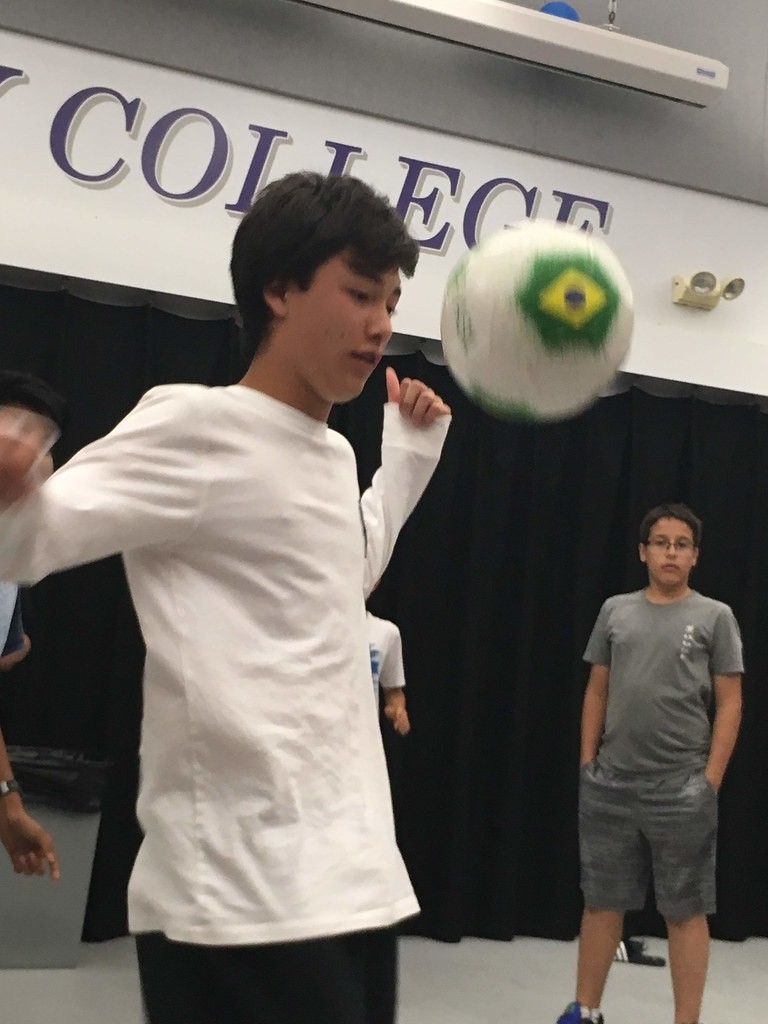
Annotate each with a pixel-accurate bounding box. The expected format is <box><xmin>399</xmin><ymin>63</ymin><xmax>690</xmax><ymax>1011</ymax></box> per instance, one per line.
<box><xmin>0</xmin><ymin>774</ymin><xmax>24</xmax><ymax>805</ymax></box>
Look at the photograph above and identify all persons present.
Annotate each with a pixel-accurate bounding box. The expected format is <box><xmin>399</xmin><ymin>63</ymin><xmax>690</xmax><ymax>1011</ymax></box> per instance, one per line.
<box><xmin>556</xmin><ymin>505</ymin><xmax>744</xmax><ymax>1024</ymax></box>
<box><xmin>1</xmin><ymin>587</ymin><xmax>64</xmax><ymax>881</ymax></box>
<box><xmin>0</xmin><ymin>172</ymin><xmax>454</xmax><ymax>1024</ymax></box>
<box><xmin>359</xmin><ymin>616</ymin><xmax>411</xmax><ymax>740</ymax></box>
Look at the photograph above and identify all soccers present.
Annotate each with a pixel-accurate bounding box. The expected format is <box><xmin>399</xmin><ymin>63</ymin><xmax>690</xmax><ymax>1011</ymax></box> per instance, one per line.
<box><xmin>440</xmin><ymin>217</ymin><xmax>639</xmax><ymax>425</ymax></box>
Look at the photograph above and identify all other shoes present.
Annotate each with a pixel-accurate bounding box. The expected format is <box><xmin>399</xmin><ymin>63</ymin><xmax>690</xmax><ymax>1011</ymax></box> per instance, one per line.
<box><xmin>555</xmin><ymin>1001</ymin><xmax>604</xmax><ymax>1024</ymax></box>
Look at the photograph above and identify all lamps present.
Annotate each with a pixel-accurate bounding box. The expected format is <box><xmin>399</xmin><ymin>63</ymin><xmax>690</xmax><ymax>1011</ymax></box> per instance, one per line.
<box><xmin>671</xmin><ymin>266</ymin><xmax>748</xmax><ymax>314</ymax></box>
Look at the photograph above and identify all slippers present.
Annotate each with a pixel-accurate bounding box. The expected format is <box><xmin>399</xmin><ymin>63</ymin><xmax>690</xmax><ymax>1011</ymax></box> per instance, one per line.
<box><xmin>613</xmin><ymin>940</ymin><xmax>667</xmax><ymax>966</ymax></box>
